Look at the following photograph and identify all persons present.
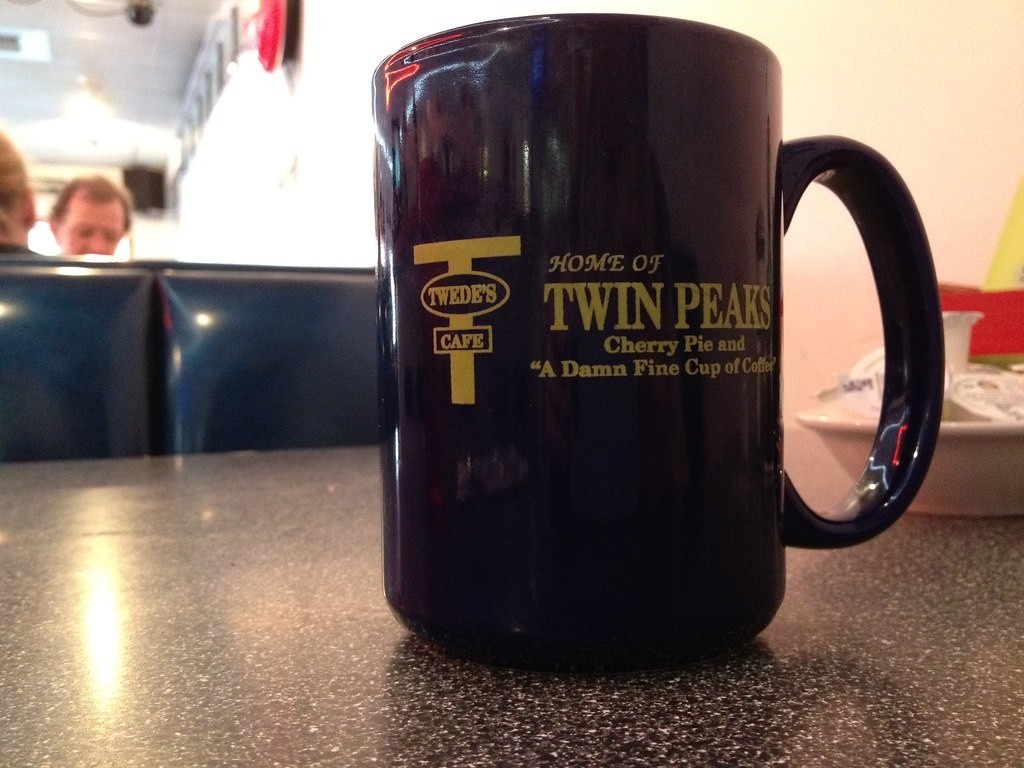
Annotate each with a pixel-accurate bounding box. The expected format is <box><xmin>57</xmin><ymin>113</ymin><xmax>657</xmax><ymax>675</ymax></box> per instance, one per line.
<box><xmin>0</xmin><ymin>130</ymin><xmax>40</xmax><ymax>254</ymax></box>
<box><xmin>47</xmin><ymin>176</ymin><xmax>132</xmax><ymax>257</ymax></box>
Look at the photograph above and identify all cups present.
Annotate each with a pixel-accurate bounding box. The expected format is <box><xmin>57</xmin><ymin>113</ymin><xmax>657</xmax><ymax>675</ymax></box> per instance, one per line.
<box><xmin>372</xmin><ymin>13</ymin><xmax>945</xmax><ymax>674</ymax></box>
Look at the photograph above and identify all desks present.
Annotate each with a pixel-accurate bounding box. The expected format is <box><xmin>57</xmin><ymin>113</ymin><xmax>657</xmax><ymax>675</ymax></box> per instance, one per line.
<box><xmin>1</xmin><ymin>415</ymin><xmax>1023</xmax><ymax>767</ymax></box>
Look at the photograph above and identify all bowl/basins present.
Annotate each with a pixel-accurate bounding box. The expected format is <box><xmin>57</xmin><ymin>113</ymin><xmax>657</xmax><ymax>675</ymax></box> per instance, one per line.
<box><xmin>797</xmin><ymin>405</ymin><xmax>1024</xmax><ymax>518</ymax></box>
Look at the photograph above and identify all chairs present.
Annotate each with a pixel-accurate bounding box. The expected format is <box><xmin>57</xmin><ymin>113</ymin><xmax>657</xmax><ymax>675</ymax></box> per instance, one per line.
<box><xmin>163</xmin><ymin>255</ymin><xmax>395</xmax><ymax>456</ymax></box>
<box><xmin>1</xmin><ymin>259</ymin><xmax>158</xmax><ymax>464</ymax></box>
